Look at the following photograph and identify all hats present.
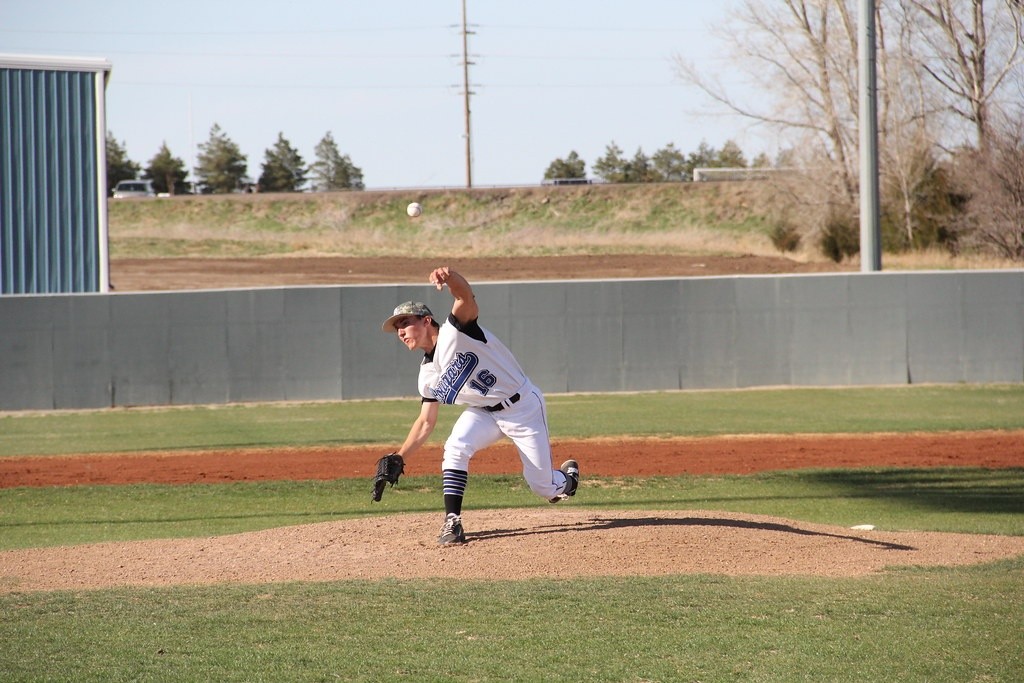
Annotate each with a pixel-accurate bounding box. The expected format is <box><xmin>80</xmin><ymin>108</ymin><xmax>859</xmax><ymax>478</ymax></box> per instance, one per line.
<box><xmin>382</xmin><ymin>301</ymin><xmax>433</xmax><ymax>333</ymax></box>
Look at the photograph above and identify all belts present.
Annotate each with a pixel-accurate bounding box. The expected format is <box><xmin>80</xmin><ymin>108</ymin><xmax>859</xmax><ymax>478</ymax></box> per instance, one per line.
<box><xmin>483</xmin><ymin>393</ymin><xmax>521</xmax><ymax>412</ymax></box>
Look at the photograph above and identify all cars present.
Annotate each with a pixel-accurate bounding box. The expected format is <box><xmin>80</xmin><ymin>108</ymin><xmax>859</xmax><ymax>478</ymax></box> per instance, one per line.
<box><xmin>111</xmin><ymin>178</ymin><xmax>159</xmax><ymax>199</ymax></box>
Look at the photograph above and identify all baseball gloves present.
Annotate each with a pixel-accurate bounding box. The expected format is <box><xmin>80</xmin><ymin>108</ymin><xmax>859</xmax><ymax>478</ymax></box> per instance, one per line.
<box><xmin>373</xmin><ymin>451</ymin><xmax>404</xmax><ymax>502</ymax></box>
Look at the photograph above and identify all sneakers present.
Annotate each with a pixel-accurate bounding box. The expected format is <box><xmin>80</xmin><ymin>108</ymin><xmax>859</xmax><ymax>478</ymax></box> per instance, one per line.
<box><xmin>440</xmin><ymin>513</ymin><xmax>465</xmax><ymax>545</ymax></box>
<box><xmin>549</xmin><ymin>460</ymin><xmax>579</xmax><ymax>504</ymax></box>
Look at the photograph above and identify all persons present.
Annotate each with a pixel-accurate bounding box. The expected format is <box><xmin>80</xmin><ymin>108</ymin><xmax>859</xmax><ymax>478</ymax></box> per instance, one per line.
<box><xmin>372</xmin><ymin>267</ymin><xmax>579</xmax><ymax>545</ymax></box>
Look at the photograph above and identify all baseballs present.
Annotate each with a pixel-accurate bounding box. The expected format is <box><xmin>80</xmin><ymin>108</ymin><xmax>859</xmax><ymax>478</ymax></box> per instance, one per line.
<box><xmin>406</xmin><ymin>202</ymin><xmax>423</xmax><ymax>217</ymax></box>
